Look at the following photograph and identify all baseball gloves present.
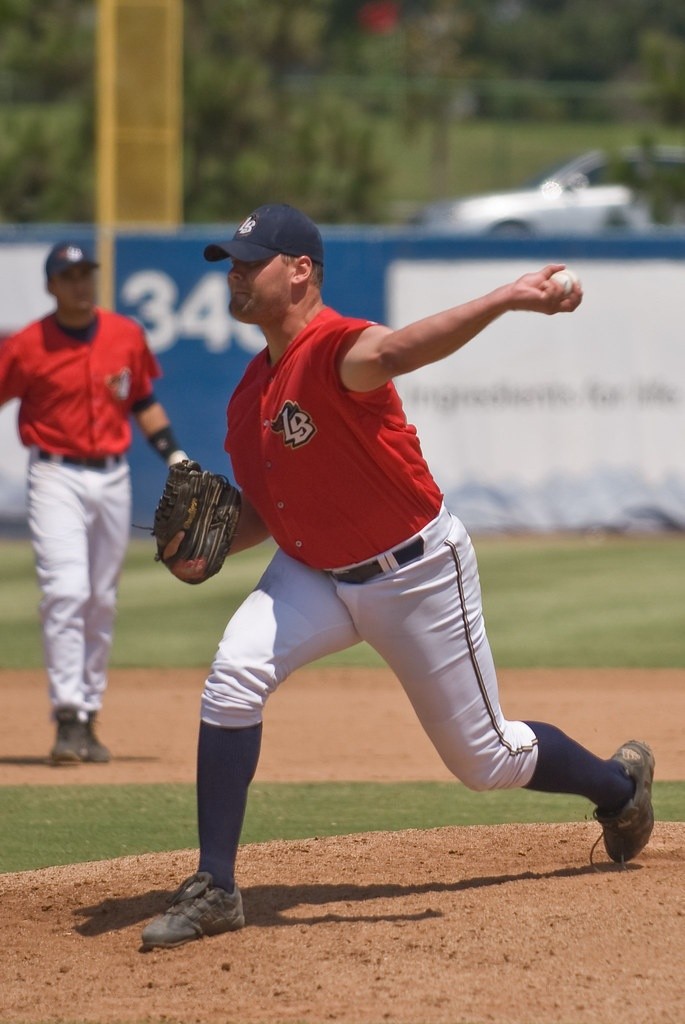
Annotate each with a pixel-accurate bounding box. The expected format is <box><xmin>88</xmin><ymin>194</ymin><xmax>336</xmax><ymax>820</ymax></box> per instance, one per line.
<box><xmin>129</xmin><ymin>458</ymin><xmax>243</xmax><ymax>586</ymax></box>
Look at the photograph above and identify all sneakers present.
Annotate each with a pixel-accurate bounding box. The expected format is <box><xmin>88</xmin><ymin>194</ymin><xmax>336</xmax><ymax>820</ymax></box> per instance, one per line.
<box><xmin>81</xmin><ymin>711</ymin><xmax>111</xmax><ymax>760</ymax></box>
<box><xmin>50</xmin><ymin>708</ymin><xmax>81</xmax><ymax>764</ymax></box>
<box><xmin>142</xmin><ymin>872</ymin><xmax>246</xmax><ymax>948</ymax></box>
<box><xmin>593</xmin><ymin>740</ymin><xmax>655</xmax><ymax>863</ymax></box>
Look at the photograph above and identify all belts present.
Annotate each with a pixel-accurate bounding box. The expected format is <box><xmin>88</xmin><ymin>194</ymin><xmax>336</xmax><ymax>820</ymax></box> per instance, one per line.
<box><xmin>40</xmin><ymin>450</ymin><xmax>120</xmax><ymax>470</ymax></box>
<box><xmin>327</xmin><ymin>537</ymin><xmax>424</xmax><ymax>584</ymax></box>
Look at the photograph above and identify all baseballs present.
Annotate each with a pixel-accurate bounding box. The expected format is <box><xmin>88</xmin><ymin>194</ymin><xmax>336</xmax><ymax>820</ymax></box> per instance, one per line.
<box><xmin>550</xmin><ymin>268</ymin><xmax>582</xmax><ymax>299</ymax></box>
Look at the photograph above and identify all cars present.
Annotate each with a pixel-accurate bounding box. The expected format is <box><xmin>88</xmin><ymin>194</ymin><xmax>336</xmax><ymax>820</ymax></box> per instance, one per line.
<box><xmin>415</xmin><ymin>140</ymin><xmax>685</xmax><ymax>231</ymax></box>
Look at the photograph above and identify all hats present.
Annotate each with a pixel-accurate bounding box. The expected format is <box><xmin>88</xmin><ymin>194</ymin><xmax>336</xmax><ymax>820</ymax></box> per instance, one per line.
<box><xmin>45</xmin><ymin>239</ymin><xmax>99</xmax><ymax>278</ymax></box>
<box><xmin>204</xmin><ymin>203</ymin><xmax>324</xmax><ymax>265</ymax></box>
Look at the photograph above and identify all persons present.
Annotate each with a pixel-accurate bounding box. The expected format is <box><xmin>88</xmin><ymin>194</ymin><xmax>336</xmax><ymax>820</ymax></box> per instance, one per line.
<box><xmin>1</xmin><ymin>236</ymin><xmax>196</xmax><ymax>768</ymax></box>
<box><xmin>135</xmin><ymin>196</ymin><xmax>656</xmax><ymax>954</ymax></box>
